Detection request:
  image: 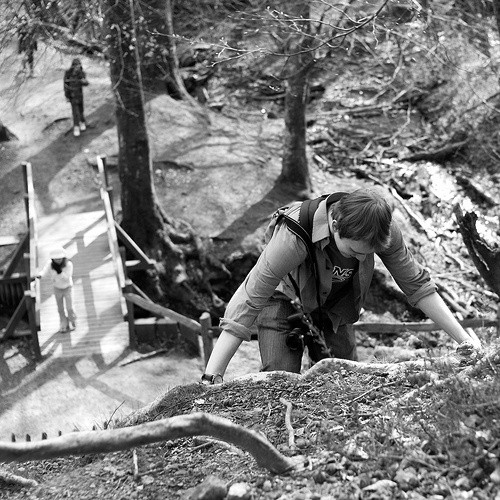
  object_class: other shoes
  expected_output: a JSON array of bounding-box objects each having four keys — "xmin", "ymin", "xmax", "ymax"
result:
[
  {"xmin": 79, "ymin": 122, "xmax": 86, "ymax": 131},
  {"xmin": 60, "ymin": 328, "xmax": 66, "ymax": 332},
  {"xmin": 70, "ymin": 320, "xmax": 75, "ymax": 326},
  {"xmin": 74, "ymin": 126, "xmax": 80, "ymax": 136}
]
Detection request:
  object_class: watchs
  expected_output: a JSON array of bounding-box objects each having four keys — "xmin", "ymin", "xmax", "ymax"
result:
[{"xmin": 202, "ymin": 374, "xmax": 224, "ymax": 384}]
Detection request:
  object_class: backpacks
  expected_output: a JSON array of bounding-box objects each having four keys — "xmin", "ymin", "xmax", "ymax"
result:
[{"xmin": 265, "ymin": 193, "xmax": 329, "ymax": 281}]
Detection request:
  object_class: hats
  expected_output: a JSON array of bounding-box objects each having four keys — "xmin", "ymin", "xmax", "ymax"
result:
[{"xmin": 50, "ymin": 248, "xmax": 66, "ymax": 258}]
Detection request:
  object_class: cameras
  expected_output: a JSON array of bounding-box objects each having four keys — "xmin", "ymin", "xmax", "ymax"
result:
[{"xmin": 286, "ymin": 313, "xmax": 318, "ymax": 352}]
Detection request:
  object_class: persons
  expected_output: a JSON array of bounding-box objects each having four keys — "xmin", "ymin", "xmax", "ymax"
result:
[
  {"xmin": 202, "ymin": 189, "xmax": 481, "ymax": 385},
  {"xmin": 16, "ymin": 29, "xmax": 39, "ymax": 78},
  {"xmin": 33, "ymin": 250, "xmax": 80, "ymax": 332},
  {"xmin": 64, "ymin": 58, "xmax": 90, "ymax": 136}
]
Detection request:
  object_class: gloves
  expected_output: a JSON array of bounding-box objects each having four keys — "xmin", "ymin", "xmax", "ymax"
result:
[{"xmin": 51, "ymin": 260, "xmax": 61, "ymax": 274}]
